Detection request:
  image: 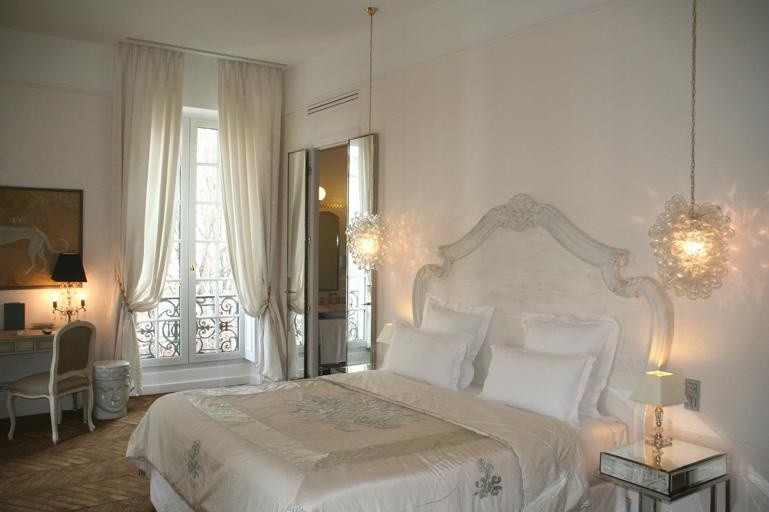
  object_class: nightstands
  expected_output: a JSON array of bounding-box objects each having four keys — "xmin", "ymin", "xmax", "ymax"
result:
[{"xmin": 593, "ymin": 436, "xmax": 732, "ymax": 512}]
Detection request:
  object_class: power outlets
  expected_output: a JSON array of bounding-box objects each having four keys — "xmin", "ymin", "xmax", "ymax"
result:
[{"xmin": 683, "ymin": 378, "xmax": 701, "ymax": 412}]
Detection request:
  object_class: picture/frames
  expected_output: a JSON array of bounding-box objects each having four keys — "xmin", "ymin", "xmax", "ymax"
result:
[{"xmin": 0, "ymin": 186, "xmax": 84, "ymax": 289}]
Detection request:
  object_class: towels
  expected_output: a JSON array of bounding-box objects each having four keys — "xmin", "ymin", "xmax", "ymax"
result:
[{"xmin": 318, "ymin": 318, "xmax": 347, "ymax": 364}]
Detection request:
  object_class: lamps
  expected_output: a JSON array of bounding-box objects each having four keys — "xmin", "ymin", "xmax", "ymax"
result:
[
  {"xmin": 376, "ymin": 324, "xmax": 394, "ymax": 343},
  {"xmin": 345, "ymin": 6, "xmax": 391, "ymax": 273},
  {"xmin": 629, "ymin": 370, "xmax": 688, "ymax": 465},
  {"xmin": 648, "ymin": 1, "xmax": 736, "ymax": 301},
  {"xmin": 50, "ymin": 252, "xmax": 87, "ymax": 410},
  {"xmin": 319, "ymin": 186, "xmax": 326, "ymax": 200}
]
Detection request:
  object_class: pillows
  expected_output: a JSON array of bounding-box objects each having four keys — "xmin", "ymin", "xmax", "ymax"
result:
[
  {"xmin": 474, "ymin": 344, "xmax": 597, "ymax": 429},
  {"xmin": 381, "ymin": 318, "xmax": 478, "ymax": 392},
  {"xmin": 520, "ymin": 311, "xmax": 620, "ymax": 421},
  {"xmin": 420, "ymin": 293, "xmax": 495, "ymax": 390}
]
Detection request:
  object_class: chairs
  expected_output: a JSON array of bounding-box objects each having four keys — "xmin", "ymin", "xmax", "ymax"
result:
[{"xmin": 6, "ymin": 321, "xmax": 96, "ymax": 445}]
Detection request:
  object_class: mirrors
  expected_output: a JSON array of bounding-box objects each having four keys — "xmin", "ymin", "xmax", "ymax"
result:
[
  {"xmin": 287, "ymin": 149, "xmax": 305, "ymax": 380},
  {"xmin": 319, "ymin": 212, "xmax": 339, "ymax": 291},
  {"xmin": 348, "ymin": 133, "xmax": 378, "ymax": 372}
]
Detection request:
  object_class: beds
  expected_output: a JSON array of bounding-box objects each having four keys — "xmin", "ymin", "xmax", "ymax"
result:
[{"xmin": 126, "ymin": 193, "xmax": 674, "ymax": 512}]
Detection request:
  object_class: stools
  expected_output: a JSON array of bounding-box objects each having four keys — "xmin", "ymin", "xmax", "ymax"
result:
[{"xmin": 93, "ymin": 360, "xmax": 132, "ymax": 420}]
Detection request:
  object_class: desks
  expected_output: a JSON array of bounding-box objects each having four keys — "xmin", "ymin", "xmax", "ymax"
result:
[{"xmin": 0, "ymin": 329, "xmax": 88, "ymax": 425}]
